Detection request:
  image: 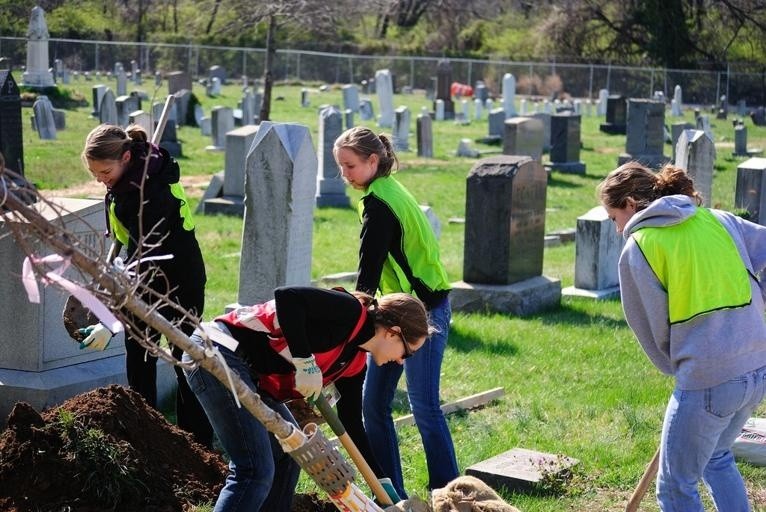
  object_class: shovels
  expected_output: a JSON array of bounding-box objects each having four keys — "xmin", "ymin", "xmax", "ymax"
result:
[{"xmin": 63, "ymin": 94, "xmax": 176, "ymax": 344}]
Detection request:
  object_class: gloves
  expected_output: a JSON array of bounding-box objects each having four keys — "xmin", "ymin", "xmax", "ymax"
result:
[
  {"xmin": 79, "ymin": 322, "xmax": 113, "ymax": 352},
  {"xmin": 292, "ymin": 354, "xmax": 323, "ymax": 401}
]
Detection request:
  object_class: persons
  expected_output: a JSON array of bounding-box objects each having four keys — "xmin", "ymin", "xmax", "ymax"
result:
[
  {"xmin": 331, "ymin": 126, "xmax": 460, "ymax": 499},
  {"xmin": 73, "ymin": 121, "xmax": 208, "ymax": 432},
  {"xmin": 597, "ymin": 159, "xmax": 766, "ymax": 511},
  {"xmin": 181, "ymin": 285, "xmax": 432, "ymax": 511}
]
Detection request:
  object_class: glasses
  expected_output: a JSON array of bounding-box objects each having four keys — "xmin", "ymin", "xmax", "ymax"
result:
[{"xmin": 401, "ymin": 331, "xmax": 414, "ymax": 359}]
